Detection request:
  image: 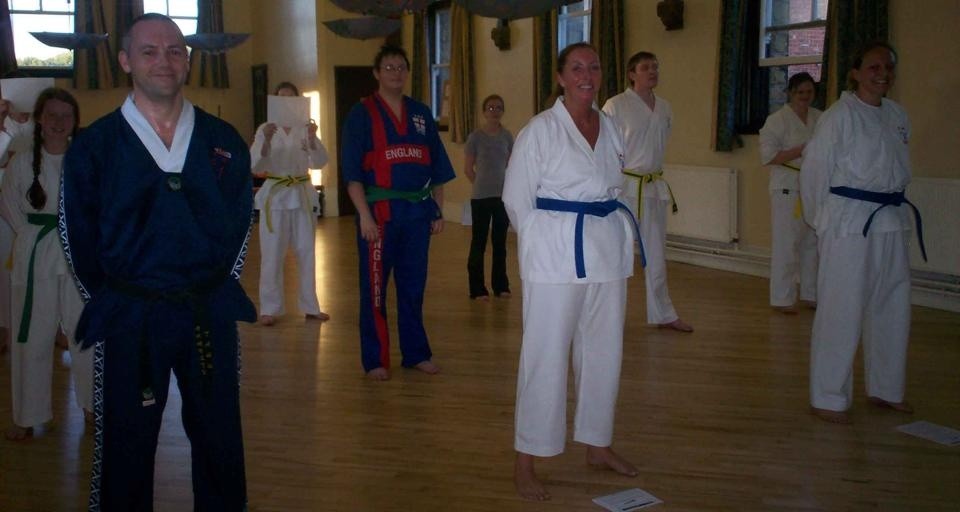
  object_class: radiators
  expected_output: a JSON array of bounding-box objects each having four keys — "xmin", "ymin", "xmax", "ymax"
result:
[
  {"xmin": 663, "ymin": 165, "xmax": 738, "ymax": 250},
  {"xmin": 904, "ymin": 176, "xmax": 960, "ymax": 293}
]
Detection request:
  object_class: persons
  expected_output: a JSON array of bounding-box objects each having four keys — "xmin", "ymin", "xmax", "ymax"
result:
[
  {"xmin": 249, "ymin": 80, "xmax": 329, "ymax": 326},
  {"xmin": 799, "ymin": 39, "xmax": 915, "ymax": 424},
  {"xmin": 603, "ymin": 52, "xmax": 695, "ymax": 334},
  {"xmin": 0, "ymin": 94, "xmax": 30, "ymax": 358},
  {"xmin": 59, "ymin": 14, "xmax": 255, "ymax": 511},
  {"xmin": 463, "ymin": 92, "xmax": 514, "ymax": 302},
  {"xmin": 345, "ymin": 42, "xmax": 457, "ymax": 371},
  {"xmin": 758, "ymin": 71, "xmax": 827, "ymax": 314},
  {"xmin": 501, "ymin": 41, "xmax": 638, "ymax": 501},
  {"xmin": 2, "ymin": 89, "xmax": 111, "ymax": 440}
]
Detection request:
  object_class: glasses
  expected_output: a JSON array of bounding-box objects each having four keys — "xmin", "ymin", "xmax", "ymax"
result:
[
  {"xmin": 379, "ymin": 63, "xmax": 406, "ymax": 73},
  {"xmin": 486, "ymin": 106, "xmax": 503, "ymax": 112}
]
